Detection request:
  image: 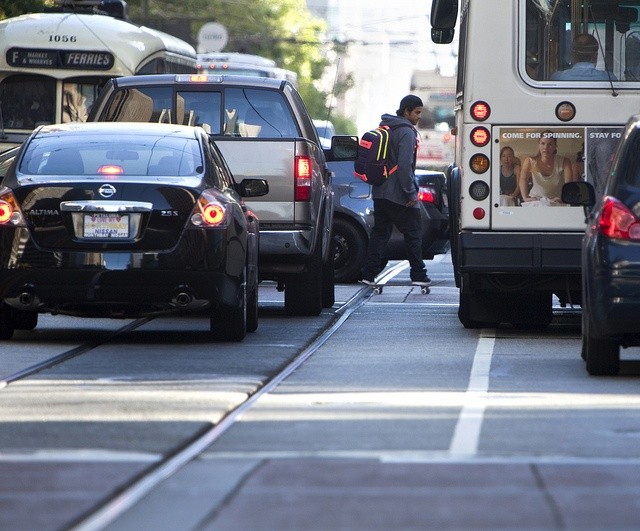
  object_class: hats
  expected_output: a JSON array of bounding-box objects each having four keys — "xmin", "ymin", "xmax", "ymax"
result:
[{"xmin": 400, "ymin": 95, "xmax": 423, "ymax": 111}]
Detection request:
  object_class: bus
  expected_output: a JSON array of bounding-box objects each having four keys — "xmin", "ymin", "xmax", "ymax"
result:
[
  {"xmin": 0, "ymin": 13, "xmax": 197, "ymax": 178},
  {"xmin": 410, "ymin": 70, "xmax": 455, "ymax": 173},
  {"xmin": 431, "ymin": 1, "xmax": 640, "ymax": 331}
]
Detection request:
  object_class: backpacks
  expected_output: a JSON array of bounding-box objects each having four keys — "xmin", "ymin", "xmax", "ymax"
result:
[{"xmin": 354, "ymin": 124, "xmax": 422, "ymax": 187}]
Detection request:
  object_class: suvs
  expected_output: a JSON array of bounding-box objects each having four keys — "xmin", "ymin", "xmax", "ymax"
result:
[{"xmin": 86, "ymin": 75, "xmax": 360, "ymax": 317}]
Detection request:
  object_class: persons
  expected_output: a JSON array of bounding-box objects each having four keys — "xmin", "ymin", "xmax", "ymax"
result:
[
  {"xmin": 517, "ymin": 129, "xmax": 573, "ymax": 207},
  {"xmin": 359, "ymin": 94, "xmax": 431, "ymax": 283},
  {"xmin": 546, "ymin": 33, "xmax": 618, "ymax": 82},
  {"xmin": 500, "ymin": 145, "xmax": 521, "ymax": 201}
]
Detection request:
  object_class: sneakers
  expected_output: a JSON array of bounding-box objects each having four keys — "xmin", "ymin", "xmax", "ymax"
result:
[
  {"xmin": 411, "ymin": 275, "xmax": 431, "ymax": 285},
  {"xmin": 362, "ymin": 274, "xmax": 375, "ymax": 285}
]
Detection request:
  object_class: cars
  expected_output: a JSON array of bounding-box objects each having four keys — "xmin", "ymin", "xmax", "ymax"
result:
[
  {"xmin": 560, "ymin": 115, "xmax": 639, "ymax": 375},
  {"xmin": 0, "ymin": 122, "xmax": 260, "ymax": 343},
  {"xmin": 322, "ymin": 146, "xmax": 449, "ymax": 284}
]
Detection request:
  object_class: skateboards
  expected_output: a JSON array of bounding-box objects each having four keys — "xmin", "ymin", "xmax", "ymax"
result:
[{"xmin": 358, "ymin": 279, "xmax": 432, "ymax": 294}]
